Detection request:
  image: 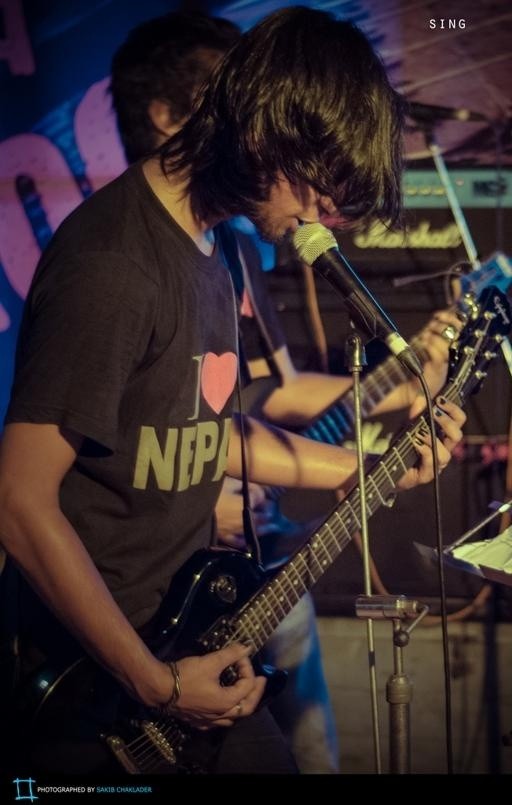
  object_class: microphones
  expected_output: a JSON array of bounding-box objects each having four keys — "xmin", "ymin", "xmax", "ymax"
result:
[
  {"xmin": 292, "ymin": 222, "xmax": 424, "ymax": 377},
  {"xmin": 354, "ymin": 594, "xmax": 428, "ymax": 647},
  {"xmin": 407, "ymin": 98, "xmax": 469, "ymax": 123}
]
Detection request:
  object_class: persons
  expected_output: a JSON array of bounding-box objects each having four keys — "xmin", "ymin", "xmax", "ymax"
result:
[
  {"xmin": 1, "ymin": 7, "xmax": 470, "ymax": 775},
  {"xmin": 102, "ymin": 13, "xmax": 462, "ymax": 775}
]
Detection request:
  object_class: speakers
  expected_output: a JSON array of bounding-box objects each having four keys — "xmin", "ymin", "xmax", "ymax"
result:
[{"xmin": 260, "ymin": 166, "xmax": 512, "ymax": 617}]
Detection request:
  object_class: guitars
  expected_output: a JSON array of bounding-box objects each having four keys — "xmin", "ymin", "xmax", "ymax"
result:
[
  {"xmin": 34, "ymin": 283, "xmax": 510, "ymax": 777},
  {"xmin": 220, "ymin": 290, "xmax": 481, "ymax": 536}
]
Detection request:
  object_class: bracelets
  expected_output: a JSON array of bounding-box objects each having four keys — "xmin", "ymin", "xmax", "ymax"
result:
[{"xmin": 152, "ymin": 660, "xmax": 182, "ymax": 715}]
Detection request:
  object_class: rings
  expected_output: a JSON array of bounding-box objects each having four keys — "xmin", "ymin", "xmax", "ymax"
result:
[
  {"xmin": 440, "ymin": 325, "xmax": 456, "ymax": 340},
  {"xmin": 236, "ymin": 702, "xmax": 243, "ymax": 714}
]
